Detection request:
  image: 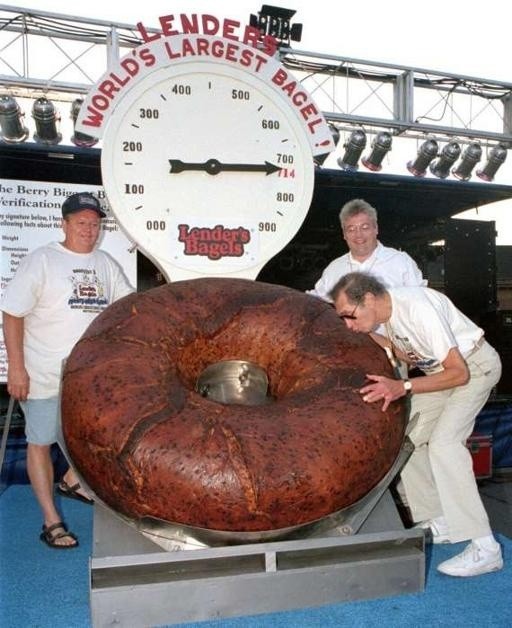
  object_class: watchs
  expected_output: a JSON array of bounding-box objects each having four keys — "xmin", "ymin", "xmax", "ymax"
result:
[{"xmin": 404, "ymin": 378, "xmax": 412, "ymax": 395}]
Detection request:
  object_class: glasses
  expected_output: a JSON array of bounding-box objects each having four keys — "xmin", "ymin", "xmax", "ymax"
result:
[{"xmin": 339, "ymin": 304, "xmax": 358, "ymax": 319}]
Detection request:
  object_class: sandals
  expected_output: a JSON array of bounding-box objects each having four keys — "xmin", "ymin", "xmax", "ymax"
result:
[
  {"xmin": 56, "ymin": 482, "xmax": 94, "ymax": 505},
  {"xmin": 40, "ymin": 522, "xmax": 79, "ymax": 548}
]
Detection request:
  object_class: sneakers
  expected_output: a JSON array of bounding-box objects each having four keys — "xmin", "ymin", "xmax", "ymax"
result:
[
  {"xmin": 437, "ymin": 543, "xmax": 503, "ymax": 577},
  {"xmin": 413, "ymin": 519, "xmax": 450, "ymax": 544}
]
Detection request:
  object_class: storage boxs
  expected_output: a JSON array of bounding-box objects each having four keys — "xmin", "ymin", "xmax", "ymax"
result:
[{"xmin": 461, "ymin": 432, "xmax": 494, "ymax": 486}]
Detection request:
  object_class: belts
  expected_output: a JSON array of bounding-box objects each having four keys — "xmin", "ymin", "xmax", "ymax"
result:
[{"xmin": 473, "ymin": 337, "xmax": 484, "ymax": 352}]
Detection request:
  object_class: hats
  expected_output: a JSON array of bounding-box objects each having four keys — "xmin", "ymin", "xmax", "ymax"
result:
[{"xmin": 62, "ymin": 192, "xmax": 106, "ymax": 217}]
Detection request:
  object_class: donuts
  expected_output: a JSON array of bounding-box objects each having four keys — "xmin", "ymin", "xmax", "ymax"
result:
[{"xmin": 61, "ymin": 277, "xmax": 407, "ymax": 532}]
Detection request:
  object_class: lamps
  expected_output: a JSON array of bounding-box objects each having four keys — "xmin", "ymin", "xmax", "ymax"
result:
[
  {"xmin": 310, "ymin": 117, "xmax": 510, "ymax": 188},
  {"xmin": 0, "ymin": 87, "xmax": 101, "ymax": 152}
]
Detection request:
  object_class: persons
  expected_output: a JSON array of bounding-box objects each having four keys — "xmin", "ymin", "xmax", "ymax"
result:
[
  {"xmin": 1, "ymin": 193, "xmax": 137, "ymax": 549},
  {"xmin": 305, "ymin": 198, "xmax": 429, "ymax": 525},
  {"xmin": 329, "ymin": 273, "xmax": 505, "ymax": 578}
]
